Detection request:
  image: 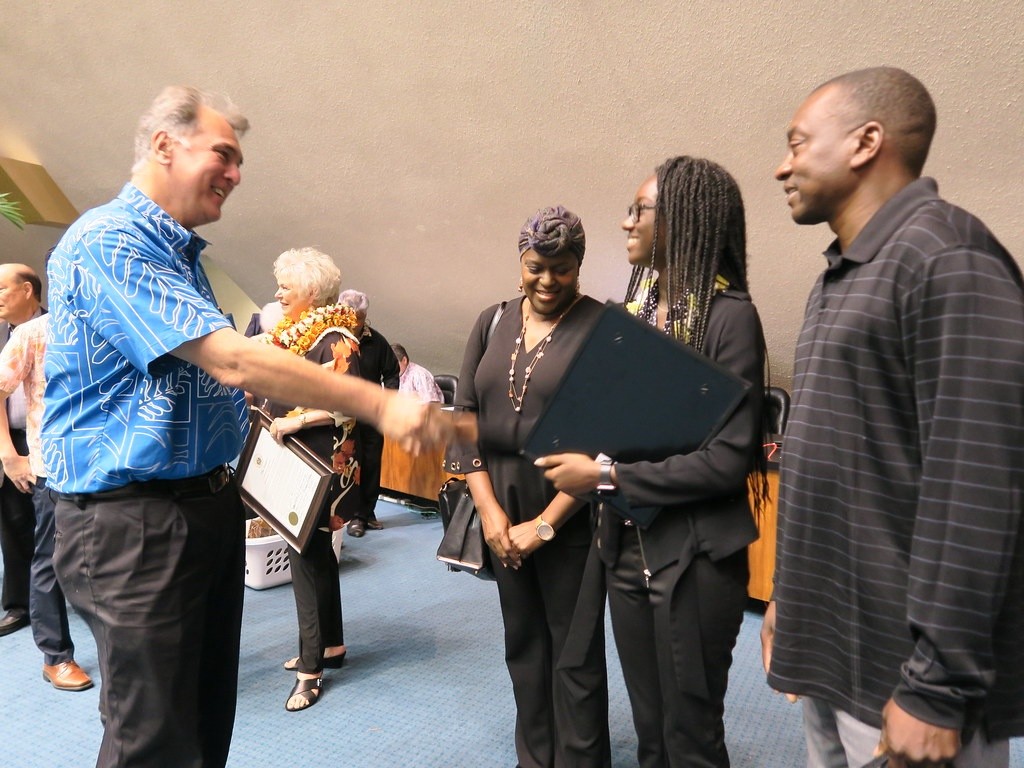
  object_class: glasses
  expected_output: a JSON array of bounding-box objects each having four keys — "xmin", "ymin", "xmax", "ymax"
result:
[{"xmin": 627, "ymin": 202, "xmax": 656, "ymax": 223}]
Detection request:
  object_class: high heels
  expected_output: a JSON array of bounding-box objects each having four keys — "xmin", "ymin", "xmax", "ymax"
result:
[
  {"xmin": 283, "ymin": 650, "xmax": 346, "ymax": 671},
  {"xmin": 285, "ymin": 669, "xmax": 324, "ymax": 712}
]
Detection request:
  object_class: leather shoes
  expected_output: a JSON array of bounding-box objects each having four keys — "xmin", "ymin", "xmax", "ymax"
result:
[
  {"xmin": 367, "ymin": 520, "xmax": 383, "ymax": 529},
  {"xmin": 43, "ymin": 659, "xmax": 93, "ymax": 691},
  {"xmin": 346, "ymin": 518, "xmax": 365, "ymax": 537},
  {"xmin": 0, "ymin": 609, "xmax": 30, "ymax": 635}
]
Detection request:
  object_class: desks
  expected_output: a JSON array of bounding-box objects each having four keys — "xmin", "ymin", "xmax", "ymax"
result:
[{"xmin": 380, "ymin": 421, "xmax": 780, "ymax": 608}]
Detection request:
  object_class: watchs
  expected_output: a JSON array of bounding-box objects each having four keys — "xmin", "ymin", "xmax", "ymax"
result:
[
  {"xmin": 535, "ymin": 515, "xmax": 556, "ymax": 541},
  {"xmin": 596, "ymin": 460, "xmax": 617, "ymax": 494}
]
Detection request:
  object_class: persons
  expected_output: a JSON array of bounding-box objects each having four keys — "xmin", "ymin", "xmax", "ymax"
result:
[
  {"xmin": 42, "ymin": 86, "xmax": 441, "ymax": 768},
  {"xmin": 391, "ymin": 343, "xmax": 444, "ymax": 405},
  {"xmin": 454, "ymin": 205, "xmax": 611, "ymax": 768},
  {"xmin": 760, "ymin": 67, "xmax": 1024, "ymax": 768},
  {"xmin": 0, "ymin": 311, "xmax": 94, "ymax": 691},
  {"xmin": 404, "ymin": 155, "xmax": 766, "ymax": 768},
  {"xmin": 338, "ymin": 290, "xmax": 400, "ymax": 537},
  {"xmin": 250, "ymin": 247, "xmax": 360, "ymax": 713},
  {"xmin": 0, "ymin": 263, "xmax": 48, "ymax": 635}
]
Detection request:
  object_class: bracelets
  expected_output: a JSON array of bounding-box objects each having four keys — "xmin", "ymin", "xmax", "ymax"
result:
[{"xmin": 299, "ymin": 414, "xmax": 308, "ymax": 429}]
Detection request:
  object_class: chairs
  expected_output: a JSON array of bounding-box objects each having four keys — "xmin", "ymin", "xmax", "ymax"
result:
[
  {"xmin": 765, "ymin": 386, "xmax": 789, "ymax": 438},
  {"xmin": 434, "ymin": 373, "xmax": 458, "ymax": 405}
]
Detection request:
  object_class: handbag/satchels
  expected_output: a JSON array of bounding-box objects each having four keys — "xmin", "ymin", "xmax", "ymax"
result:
[
  {"xmin": 436, "ymin": 487, "xmax": 497, "ymax": 581},
  {"xmin": 437, "ymin": 477, "xmax": 467, "ymax": 573}
]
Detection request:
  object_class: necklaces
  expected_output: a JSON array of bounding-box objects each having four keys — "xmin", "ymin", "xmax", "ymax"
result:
[
  {"xmin": 261, "ymin": 304, "xmax": 357, "ymax": 357},
  {"xmin": 509, "ymin": 312, "xmax": 565, "ymax": 412}
]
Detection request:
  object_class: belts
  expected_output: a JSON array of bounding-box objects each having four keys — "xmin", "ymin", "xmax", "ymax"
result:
[{"xmin": 58, "ymin": 464, "xmax": 229, "ymax": 501}]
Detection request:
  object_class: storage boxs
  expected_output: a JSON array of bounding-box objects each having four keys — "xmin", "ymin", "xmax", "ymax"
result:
[{"xmin": 244, "ymin": 515, "xmax": 351, "ymax": 589}]
